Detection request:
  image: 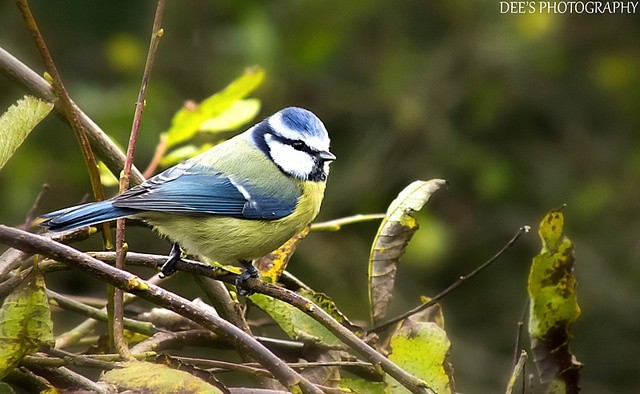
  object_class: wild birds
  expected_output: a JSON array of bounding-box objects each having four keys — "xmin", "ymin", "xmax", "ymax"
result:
[{"xmin": 40, "ymin": 106, "xmax": 339, "ymax": 297}]
